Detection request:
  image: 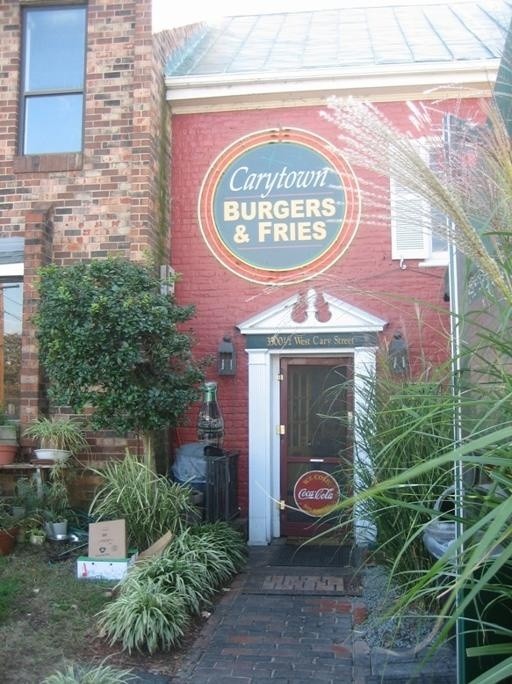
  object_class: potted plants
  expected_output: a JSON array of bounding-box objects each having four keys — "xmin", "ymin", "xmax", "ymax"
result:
[{"xmin": 0, "ymin": 406, "xmax": 96, "ymax": 556}]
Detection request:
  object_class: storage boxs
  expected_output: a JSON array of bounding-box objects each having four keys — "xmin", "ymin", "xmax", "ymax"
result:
[{"xmin": 77, "ymin": 518, "xmax": 139, "ymax": 581}]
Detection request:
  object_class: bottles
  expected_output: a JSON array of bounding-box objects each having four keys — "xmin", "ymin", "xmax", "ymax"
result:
[{"xmin": 198, "ymin": 383, "xmax": 224, "ymax": 447}]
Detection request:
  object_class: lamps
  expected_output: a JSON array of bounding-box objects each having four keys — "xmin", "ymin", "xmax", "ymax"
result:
[
  {"xmin": 388, "ymin": 331, "xmax": 409, "ymax": 373},
  {"xmin": 217, "ymin": 335, "xmax": 237, "ymax": 376}
]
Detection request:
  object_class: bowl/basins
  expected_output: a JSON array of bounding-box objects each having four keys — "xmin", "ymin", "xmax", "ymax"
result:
[{"xmin": 47, "ymin": 534, "xmax": 89, "ymax": 546}]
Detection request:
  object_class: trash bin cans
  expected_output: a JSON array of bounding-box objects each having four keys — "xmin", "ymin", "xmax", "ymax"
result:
[{"xmin": 175, "ymin": 442, "xmax": 227, "ymax": 526}]
